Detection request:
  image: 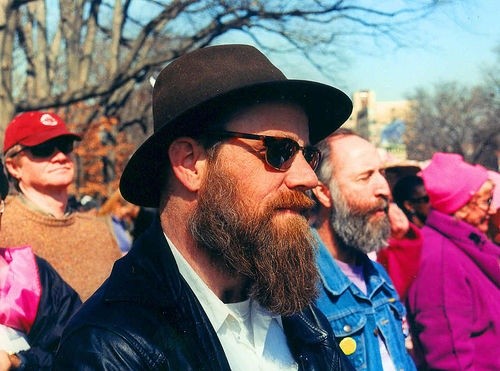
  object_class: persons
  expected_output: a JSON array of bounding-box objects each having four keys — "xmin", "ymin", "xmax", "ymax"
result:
[
  {"xmin": 306, "ymin": 127, "xmax": 417, "ymax": 371},
  {"xmin": 90, "ymin": 155, "xmax": 431, "ymax": 299},
  {"xmin": 0, "ymin": 112, "xmax": 122, "ymax": 304},
  {"xmin": 54, "ymin": 44, "xmax": 358, "ymax": 371},
  {"xmin": 0, "ymin": 158, "xmax": 84, "ymax": 371},
  {"xmin": 408, "ymin": 152, "xmax": 500, "ymax": 371}
]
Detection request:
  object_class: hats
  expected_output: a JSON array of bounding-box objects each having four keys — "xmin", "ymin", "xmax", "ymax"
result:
[
  {"xmin": 416, "ymin": 152, "xmax": 488, "ymax": 215},
  {"xmin": 2, "ymin": 111, "xmax": 82, "ymax": 153},
  {"xmin": 119, "ymin": 44, "xmax": 353, "ymax": 208}
]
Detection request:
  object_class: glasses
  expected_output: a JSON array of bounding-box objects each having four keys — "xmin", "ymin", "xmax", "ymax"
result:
[
  {"xmin": 9, "ymin": 136, "xmax": 73, "ymax": 158},
  {"xmin": 202, "ymin": 130, "xmax": 321, "ymax": 172},
  {"xmin": 409, "ymin": 194, "xmax": 430, "ymax": 204}
]
